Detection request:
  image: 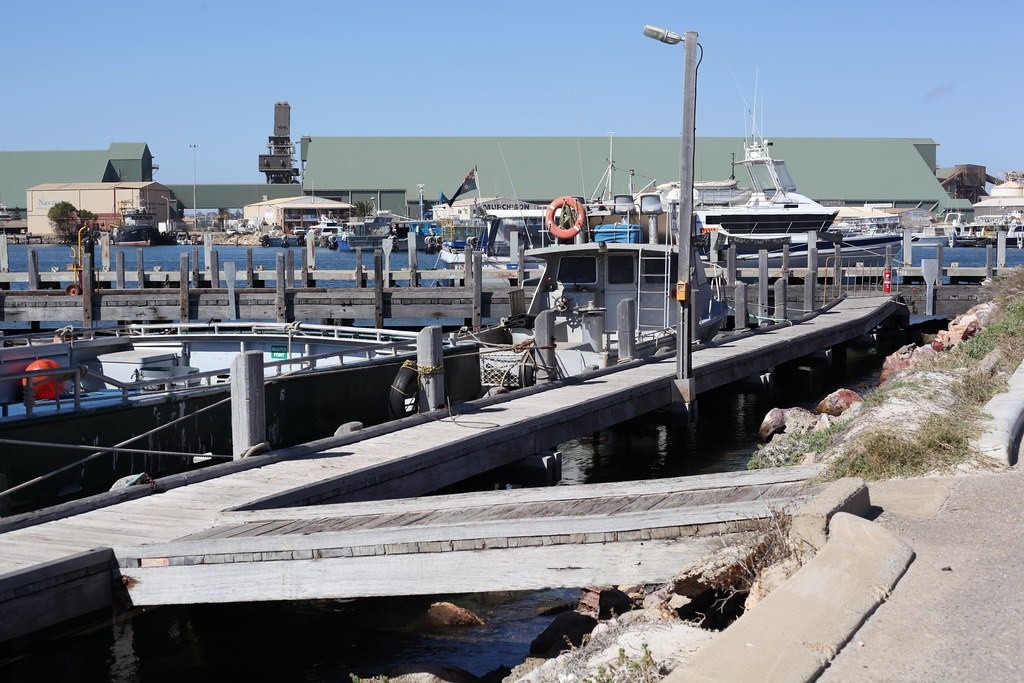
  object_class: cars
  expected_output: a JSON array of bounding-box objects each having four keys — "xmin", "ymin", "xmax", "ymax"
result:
[{"xmin": 226, "ymin": 229, "xmax": 236, "ymax": 235}]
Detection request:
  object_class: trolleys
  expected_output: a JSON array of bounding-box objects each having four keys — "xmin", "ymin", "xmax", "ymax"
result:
[{"xmin": 66, "ymin": 221, "xmax": 87, "ymax": 297}]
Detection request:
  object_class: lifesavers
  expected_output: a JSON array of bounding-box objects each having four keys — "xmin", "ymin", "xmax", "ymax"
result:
[
  {"xmin": 546, "ymin": 195, "xmax": 586, "ymax": 237},
  {"xmin": 388, "ymin": 362, "xmax": 421, "ymax": 417}
]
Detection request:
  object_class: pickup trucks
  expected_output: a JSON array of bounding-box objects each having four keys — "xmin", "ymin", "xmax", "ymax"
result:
[
  {"xmin": 272, "ymin": 225, "xmax": 283, "ymax": 233},
  {"xmin": 291, "ymin": 227, "xmax": 305, "ymax": 235}
]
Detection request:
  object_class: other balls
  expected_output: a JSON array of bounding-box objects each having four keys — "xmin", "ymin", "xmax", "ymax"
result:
[{"xmin": 24, "ymin": 359, "xmax": 64, "ymax": 401}]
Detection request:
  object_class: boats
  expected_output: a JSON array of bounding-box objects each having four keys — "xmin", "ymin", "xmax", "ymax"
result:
[
  {"xmin": 900, "ymin": 212, "xmax": 970, "ymax": 247},
  {"xmin": 961, "ymin": 210, "xmax": 1024, "ymax": 249},
  {"xmin": 0, "ymin": 322, "xmax": 482, "ymax": 517},
  {"xmin": 109, "ymin": 200, "xmax": 160, "ymax": 246},
  {"xmin": 446, "ymin": 136, "xmax": 731, "ymax": 398},
  {"xmin": 439, "ymin": 140, "xmax": 920, "ymax": 286},
  {"xmin": 260, "ymin": 215, "xmax": 439, "ymax": 253},
  {"xmin": 176, "ymin": 232, "xmax": 188, "ymax": 246}
]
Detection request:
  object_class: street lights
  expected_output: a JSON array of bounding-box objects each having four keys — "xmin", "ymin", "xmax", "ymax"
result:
[
  {"xmin": 417, "ymin": 184, "xmax": 425, "ymax": 220},
  {"xmin": 643, "ymin": 26, "xmax": 697, "ymax": 381},
  {"xmin": 161, "ymin": 196, "xmax": 170, "ymax": 231},
  {"xmin": 190, "ymin": 144, "xmax": 200, "ymax": 229}
]
